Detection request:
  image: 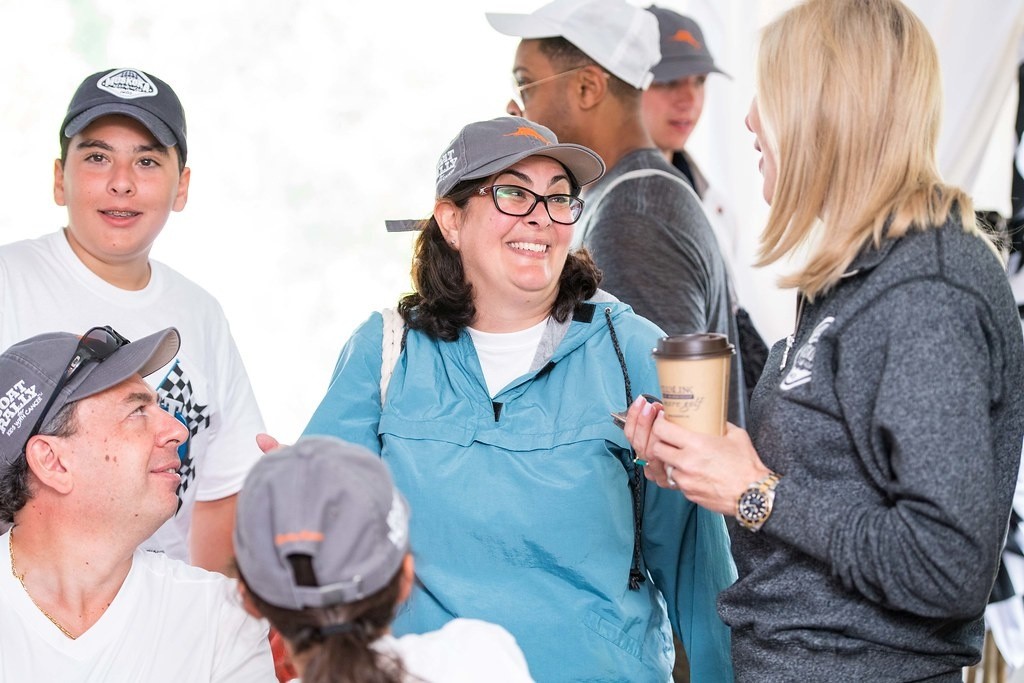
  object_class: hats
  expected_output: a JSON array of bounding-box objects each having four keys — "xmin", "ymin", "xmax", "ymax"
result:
[
  {"xmin": 643, "ymin": 4, "xmax": 730, "ymax": 84},
  {"xmin": 0, "ymin": 328, "xmax": 184, "ymax": 479},
  {"xmin": 436, "ymin": 117, "xmax": 606, "ymax": 199},
  {"xmin": 486, "ymin": 0, "xmax": 663, "ymax": 91},
  {"xmin": 232, "ymin": 435, "xmax": 409, "ymax": 610},
  {"xmin": 60, "ymin": 69, "xmax": 188, "ymax": 168}
]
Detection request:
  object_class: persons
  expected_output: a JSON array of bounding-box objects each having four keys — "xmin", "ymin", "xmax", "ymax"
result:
[
  {"xmin": 0, "ymin": 326, "xmax": 278, "ymax": 682},
  {"xmin": 486, "ymin": 0, "xmax": 747, "ymax": 429},
  {"xmin": 640, "ymin": 6, "xmax": 768, "ymax": 386},
  {"xmin": 232, "ymin": 436, "xmax": 532, "ymax": 682},
  {"xmin": 256, "ymin": 117, "xmax": 741, "ymax": 683},
  {"xmin": 624, "ymin": 0, "xmax": 1024, "ymax": 683},
  {"xmin": 1, "ymin": 66, "xmax": 267, "ymax": 576}
]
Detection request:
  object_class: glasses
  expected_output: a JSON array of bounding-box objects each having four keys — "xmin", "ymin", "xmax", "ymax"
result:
[
  {"xmin": 511, "ymin": 65, "xmax": 610, "ymax": 112},
  {"xmin": 454, "ymin": 186, "xmax": 584, "ymax": 225},
  {"xmin": 21, "ymin": 325, "xmax": 131, "ymax": 455}
]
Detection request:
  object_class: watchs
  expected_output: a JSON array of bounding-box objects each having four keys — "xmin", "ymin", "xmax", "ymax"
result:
[{"xmin": 736, "ymin": 472, "xmax": 783, "ymax": 532}]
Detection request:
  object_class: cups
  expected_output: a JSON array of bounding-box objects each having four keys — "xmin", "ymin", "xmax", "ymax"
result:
[{"xmin": 651, "ymin": 332, "xmax": 737, "ymax": 444}]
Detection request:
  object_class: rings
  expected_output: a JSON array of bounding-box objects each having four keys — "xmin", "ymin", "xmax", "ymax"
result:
[{"xmin": 667, "ymin": 466, "xmax": 677, "ymax": 488}]
